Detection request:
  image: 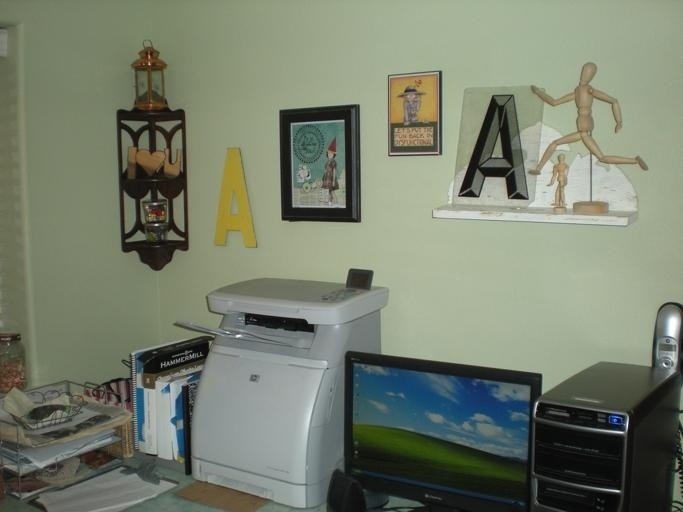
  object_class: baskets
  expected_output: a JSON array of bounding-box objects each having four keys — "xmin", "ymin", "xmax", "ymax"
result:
[{"xmin": 84, "ymin": 361, "xmax": 133, "ymax": 458}]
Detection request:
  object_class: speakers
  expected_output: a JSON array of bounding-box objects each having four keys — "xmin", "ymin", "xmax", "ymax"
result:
[{"xmin": 325, "ymin": 468, "xmax": 387, "ymax": 512}]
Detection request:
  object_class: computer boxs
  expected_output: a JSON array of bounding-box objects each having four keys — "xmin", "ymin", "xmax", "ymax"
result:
[{"xmin": 530, "ymin": 361, "xmax": 678, "ymax": 511}]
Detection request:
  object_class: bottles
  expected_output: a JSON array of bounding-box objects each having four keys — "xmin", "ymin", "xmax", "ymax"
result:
[
  {"xmin": 142, "ymin": 199, "xmax": 169, "ymax": 222},
  {"xmin": 143, "ymin": 223, "xmax": 168, "ymax": 243},
  {"xmin": 0, "ymin": 333, "xmax": 28, "ymax": 392}
]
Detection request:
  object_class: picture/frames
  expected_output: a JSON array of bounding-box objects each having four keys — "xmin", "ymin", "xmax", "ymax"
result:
[
  {"xmin": 277, "ymin": 101, "xmax": 360, "ymax": 225},
  {"xmin": 385, "ymin": 68, "xmax": 445, "ymax": 158}
]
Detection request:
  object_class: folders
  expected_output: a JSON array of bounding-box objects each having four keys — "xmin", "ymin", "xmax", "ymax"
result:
[{"xmin": 28, "ymin": 457, "xmax": 180, "ymax": 512}]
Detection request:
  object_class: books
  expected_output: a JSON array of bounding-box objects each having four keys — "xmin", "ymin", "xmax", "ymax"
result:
[
  {"xmin": 131, "ymin": 335, "xmax": 212, "ymax": 473},
  {"xmin": 34, "ymin": 465, "xmax": 180, "ymax": 512},
  {"xmin": 0, "ymin": 386, "xmax": 132, "ymax": 501}
]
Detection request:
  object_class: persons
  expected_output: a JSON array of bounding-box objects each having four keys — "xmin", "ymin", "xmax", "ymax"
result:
[
  {"xmin": 397, "ymin": 84, "xmax": 426, "ymax": 126},
  {"xmin": 531, "ymin": 62, "xmax": 648, "ymax": 176},
  {"xmin": 321, "ymin": 136, "xmax": 339, "ymax": 205},
  {"xmin": 545, "ymin": 155, "xmax": 570, "ymax": 211}
]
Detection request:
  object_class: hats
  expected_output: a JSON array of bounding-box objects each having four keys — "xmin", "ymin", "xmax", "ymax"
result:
[
  {"xmin": 328, "ymin": 137, "xmax": 336, "ymax": 153},
  {"xmin": 396, "ymin": 87, "xmax": 425, "ymax": 96}
]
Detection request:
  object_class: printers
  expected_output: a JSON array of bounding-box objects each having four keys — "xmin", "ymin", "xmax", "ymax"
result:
[{"xmin": 174, "ymin": 269, "xmax": 388, "ymax": 508}]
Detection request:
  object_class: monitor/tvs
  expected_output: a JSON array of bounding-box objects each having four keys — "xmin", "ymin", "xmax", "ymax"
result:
[{"xmin": 345, "ymin": 350, "xmax": 543, "ymax": 512}]
